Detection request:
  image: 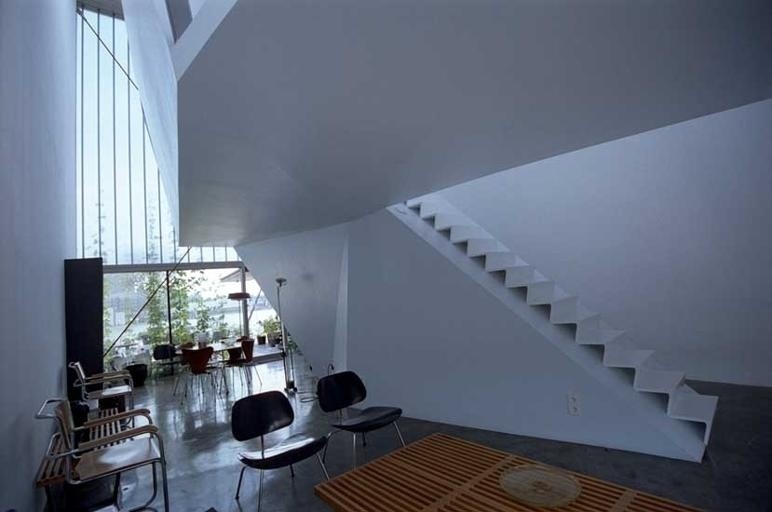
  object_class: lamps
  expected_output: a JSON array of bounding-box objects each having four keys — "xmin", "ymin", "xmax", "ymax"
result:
[{"xmin": 228, "ymin": 293, "xmax": 251, "ymax": 337}]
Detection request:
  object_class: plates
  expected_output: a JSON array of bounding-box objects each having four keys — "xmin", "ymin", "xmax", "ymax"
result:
[{"xmin": 500, "ymin": 463, "xmax": 582, "ymax": 508}]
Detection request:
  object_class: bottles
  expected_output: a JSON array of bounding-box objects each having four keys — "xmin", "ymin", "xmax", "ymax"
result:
[{"xmin": 229, "ymin": 328, "xmax": 235, "ymax": 346}]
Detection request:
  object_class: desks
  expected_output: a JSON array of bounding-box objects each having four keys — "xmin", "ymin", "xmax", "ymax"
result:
[{"xmin": 313, "ymin": 430, "xmax": 697, "ymax": 512}]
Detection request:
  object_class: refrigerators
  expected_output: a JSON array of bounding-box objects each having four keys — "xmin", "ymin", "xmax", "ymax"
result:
[{"xmin": 64, "ymin": 257, "xmax": 104, "ymax": 400}]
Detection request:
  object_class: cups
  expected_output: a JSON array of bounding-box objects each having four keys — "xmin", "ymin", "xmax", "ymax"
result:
[{"xmin": 198, "ymin": 333, "xmax": 208, "ymax": 348}]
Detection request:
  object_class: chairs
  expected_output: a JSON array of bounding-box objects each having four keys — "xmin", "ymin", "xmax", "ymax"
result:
[
  {"xmin": 231, "ymin": 390, "xmax": 330, "ymax": 512},
  {"xmin": 152, "ymin": 336, "xmax": 262, "ymax": 405},
  {"xmin": 36, "ymin": 398, "xmax": 170, "ymax": 512},
  {"xmin": 317, "ymin": 370, "xmax": 406, "ymax": 472},
  {"xmin": 69, "ymin": 361, "xmax": 135, "ymax": 428}
]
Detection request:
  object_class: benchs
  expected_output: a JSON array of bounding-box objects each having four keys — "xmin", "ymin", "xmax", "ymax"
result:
[{"xmin": 36, "ymin": 408, "xmax": 124, "ymax": 512}]
